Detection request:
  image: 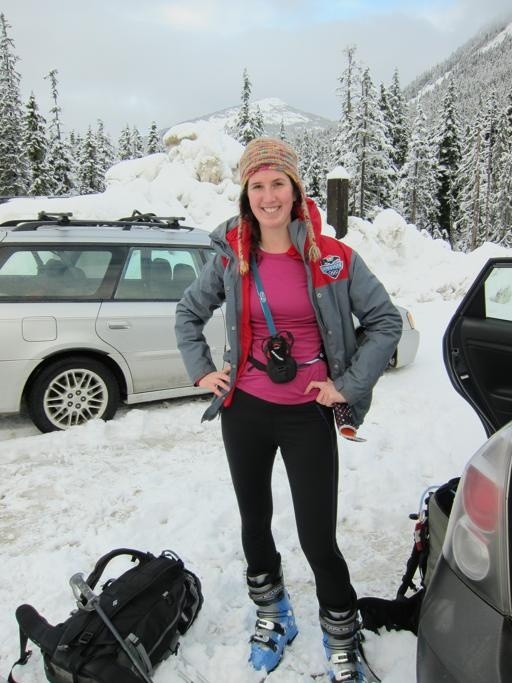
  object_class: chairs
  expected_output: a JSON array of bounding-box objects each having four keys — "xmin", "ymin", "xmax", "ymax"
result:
[
  {"xmin": 172, "ymin": 260, "xmax": 198, "ymax": 299},
  {"xmin": 145, "ymin": 257, "xmax": 174, "ymax": 301}
]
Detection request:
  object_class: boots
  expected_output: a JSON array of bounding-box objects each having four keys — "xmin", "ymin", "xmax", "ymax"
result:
[
  {"xmin": 314, "ymin": 582, "xmax": 371, "ymax": 683},
  {"xmin": 243, "ymin": 551, "xmax": 301, "ymax": 676}
]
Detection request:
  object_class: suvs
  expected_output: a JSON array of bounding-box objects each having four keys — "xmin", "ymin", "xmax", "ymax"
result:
[{"xmin": 0, "ymin": 209, "xmax": 418, "ymax": 433}]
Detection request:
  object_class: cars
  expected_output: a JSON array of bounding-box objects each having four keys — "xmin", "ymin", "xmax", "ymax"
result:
[{"xmin": 413, "ymin": 254, "xmax": 512, "ymax": 682}]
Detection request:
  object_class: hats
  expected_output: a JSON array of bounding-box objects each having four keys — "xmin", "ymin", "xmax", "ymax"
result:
[{"xmin": 234, "ymin": 135, "xmax": 324, "ymax": 277}]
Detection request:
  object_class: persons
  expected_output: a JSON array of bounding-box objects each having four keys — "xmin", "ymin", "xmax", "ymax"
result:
[{"xmin": 173, "ymin": 135, "xmax": 404, "ymax": 683}]
